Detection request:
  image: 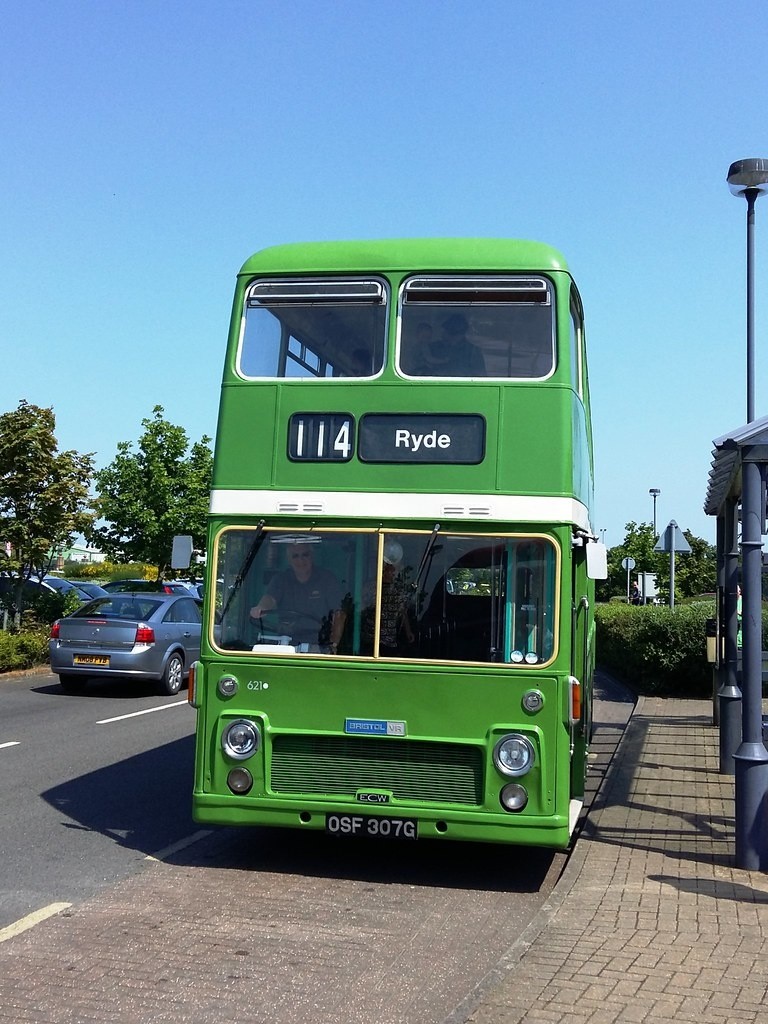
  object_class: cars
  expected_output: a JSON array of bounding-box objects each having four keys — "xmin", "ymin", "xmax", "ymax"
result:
[
  {"xmin": 0, "ymin": 574, "xmax": 110, "ymax": 615},
  {"xmin": 70, "ymin": 580, "xmax": 127, "ymax": 614},
  {"xmin": 48, "ymin": 592, "xmax": 221, "ymax": 695},
  {"xmin": 103, "ymin": 579, "xmax": 193, "ymax": 595}
]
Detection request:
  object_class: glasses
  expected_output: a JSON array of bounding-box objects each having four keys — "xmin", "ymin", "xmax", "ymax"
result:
[{"xmin": 291, "ymin": 550, "xmax": 312, "ymax": 559}]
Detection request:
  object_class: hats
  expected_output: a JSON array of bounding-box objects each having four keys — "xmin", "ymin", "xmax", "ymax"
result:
[
  {"xmin": 441, "ymin": 313, "xmax": 469, "ymax": 331},
  {"xmin": 384, "ymin": 541, "xmax": 404, "ymax": 564}
]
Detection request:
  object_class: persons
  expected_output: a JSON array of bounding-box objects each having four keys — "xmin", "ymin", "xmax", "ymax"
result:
[
  {"xmin": 363, "ymin": 565, "xmax": 414, "ymax": 655},
  {"xmin": 630, "ymin": 581, "xmax": 640, "ymax": 605},
  {"xmin": 249, "ymin": 542, "xmax": 349, "ymax": 652},
  {"xmin": 407, "ymin": 314, "xmax": 487, "ymax": 376}
]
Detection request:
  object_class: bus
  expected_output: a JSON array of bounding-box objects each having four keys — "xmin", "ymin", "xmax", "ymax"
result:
[{"xmin": 170, "ymin": 235, "xmax": 608, "ymax": 852}]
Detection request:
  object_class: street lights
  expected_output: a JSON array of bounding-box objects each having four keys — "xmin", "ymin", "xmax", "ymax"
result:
[
  {"xmin": 727, "ymin": 157, "xmax": 768, "ymax": 423},
  {"xmin": 649, "ymin": 488, "xmax": 661, "ymax": 537}
]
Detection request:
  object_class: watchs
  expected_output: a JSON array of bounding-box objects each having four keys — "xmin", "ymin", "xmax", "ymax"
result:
[{"xmin": 330, "ymin": 642, "xmax": 339, "ymax": 648}]
